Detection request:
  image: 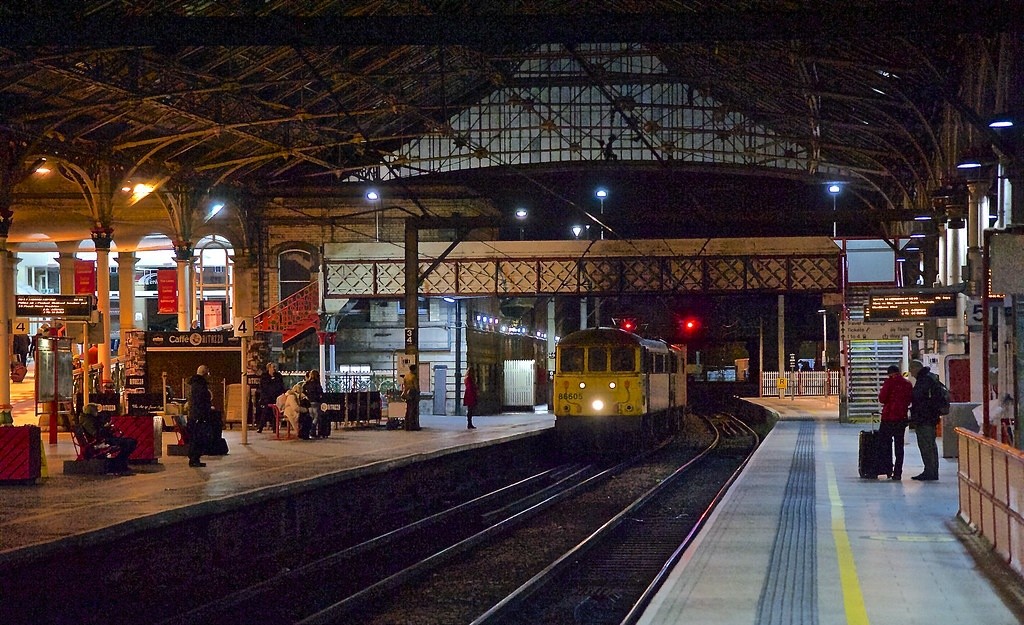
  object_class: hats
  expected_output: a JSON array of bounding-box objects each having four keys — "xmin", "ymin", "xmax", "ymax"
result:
[
  {"xmin": 82, "ymin": 403, "xmax": 98, "ymax": 415},
  {"xmin": 73, "ymin": 355, "xmax": 81, "ymax": 361},
  {"xmin": 409, "ymin": 364, "xmax": 417, "ymax": 372},
  {"xmin": 887, "ymin": 366, "xmax": 900, "ymax": 374},
  {"xmin": 196, "ymin": 365, "xmax": 209, "ymax": 376}
]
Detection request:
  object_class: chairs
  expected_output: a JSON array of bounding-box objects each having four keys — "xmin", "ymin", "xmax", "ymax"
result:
[
  {"xmin": 172, "ymin": 415, "xmax": 189, "ymax": 445},
  {"xmin": 268, "ymin": 403, "xmax": 292, "ymax": 439},
  {"xmin": 69, "ymin": 426, "xmax": 124, "ymax": 477}
]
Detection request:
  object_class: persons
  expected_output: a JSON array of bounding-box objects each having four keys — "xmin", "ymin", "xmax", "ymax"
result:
[
  {"xmin": 878, "ymin": 365, "xmax": 913, "ymax": 481},
  {"xmin": 88, "ymin": 344, "xmax": 98, "ymax": 394},
  {"xmin": 79, "ymin": 403, "xmax": 137, "ymax": 470},
  {"xmin": 29, "ymin": 328, "xmax": 44, "ymax": 358},
  {"xmin": 254, "ymin": 362, "xmax": 284, "ymax": 433},
  {"xmin": 463, "ymin": 366, "xmax": 479, "ymax": 429},
  {"xmin": 404, "ymin": 364, "xmax": 422, "ymax": 431},
  {"xmin": 908, "ymin": 359, "xmax": 939, "ymax": 482},
  {"xmin": 284, "ymin": 369, "xmax": 323, "ymax": 440},
  {"xmin": 190, "ymin": 319, "xmax": 200, "ymax": 332},
  {"xmin": 13, "ymin": 334, "xmax": 30, "ymax": 366},
  {"xmin": 188, "ymin": 365, "xmax": 211, "ymax": 467}
]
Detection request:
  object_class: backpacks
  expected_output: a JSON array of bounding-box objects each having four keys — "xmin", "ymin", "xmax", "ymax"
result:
[{"xmin": 924, "ymin": 374, "xmax": 950, "ymax": 416}]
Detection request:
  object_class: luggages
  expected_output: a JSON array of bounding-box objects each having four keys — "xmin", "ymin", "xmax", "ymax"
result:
[
  {"xmin": 10, "ymin": 357, "xmax": 31, "ymax": 384},
  {"xmin": 858, "ymin": 411, "xmax": 891, "ymax": 478}
]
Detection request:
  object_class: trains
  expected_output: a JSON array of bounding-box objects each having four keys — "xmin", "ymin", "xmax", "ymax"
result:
[{"xmin": 550, "ymin": 326, "xmax": 689, "ymax": 454}]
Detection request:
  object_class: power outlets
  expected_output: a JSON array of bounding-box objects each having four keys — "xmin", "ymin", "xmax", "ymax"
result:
[{"xmin": 956, "ymin": 156, "xmax": 1008, "ymax": 169}]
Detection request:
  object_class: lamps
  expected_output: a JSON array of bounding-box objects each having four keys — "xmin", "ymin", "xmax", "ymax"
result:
[{"xmin": 989, "ymin": 118, "xmax": 1024, "ymax": 127}]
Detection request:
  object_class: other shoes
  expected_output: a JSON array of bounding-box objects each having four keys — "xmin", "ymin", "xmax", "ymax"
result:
[
  {"xmin": 911, "ymin": 473, "xmax": 939, "ymax": 480},
  {"xmin": 273, "ymin": 430, "xmax": 276, "ymax": 433},
  {"xmin": 404, "ymin": 428, "xmax": 410, "ymax": 431},
  {"xmin": 409, "ymin": 427, "xmax": 422, "ymax": 431},
  {"xmin": 189, "ymin": 461, "xmax": 207, "ymax": 467},
  {"xmin": 256, "ymin": 428, "xmax": 262, "ymax": 433},
  {"xmin": 892, "ymin": 475, "xmax": 902, "ymax": 480}
]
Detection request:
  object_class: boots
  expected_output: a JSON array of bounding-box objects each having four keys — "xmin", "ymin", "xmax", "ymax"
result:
[{"xmin": 467, "ymin": 419, "xmax": 477, "ymax": 429}]
